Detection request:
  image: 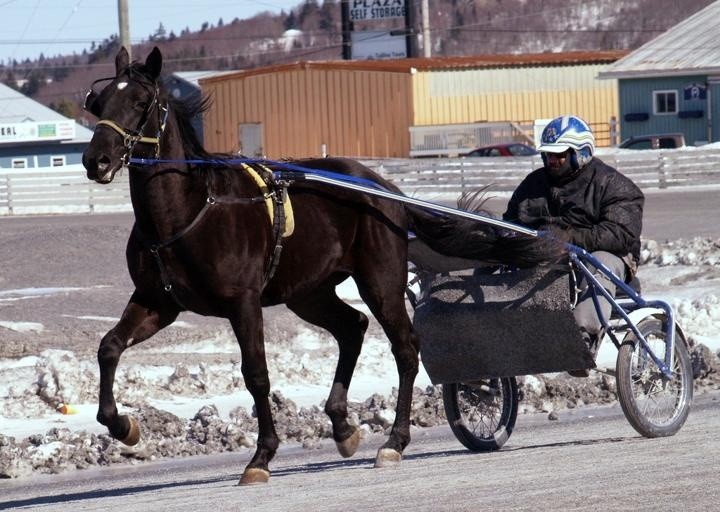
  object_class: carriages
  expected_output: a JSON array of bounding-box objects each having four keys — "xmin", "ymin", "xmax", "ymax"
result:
[{"xmin": 80, "ymin": 45, "xmax": 695, "ymax": 488}]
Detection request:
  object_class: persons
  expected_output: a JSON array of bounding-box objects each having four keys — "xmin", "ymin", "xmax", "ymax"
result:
[{"xmin": 501, "ymin": 113, "xmax": 645, "ymax": 378}]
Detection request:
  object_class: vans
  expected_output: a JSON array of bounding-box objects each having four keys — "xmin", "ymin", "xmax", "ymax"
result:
[{"xmin": 616, "ymin": 134, "xmax": 687, "ymax": 148}]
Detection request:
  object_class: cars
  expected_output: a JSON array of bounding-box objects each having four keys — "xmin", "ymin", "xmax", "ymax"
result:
[{"xmin": 461, "ymin": 142, "xmax": 544, "ymax": 158}]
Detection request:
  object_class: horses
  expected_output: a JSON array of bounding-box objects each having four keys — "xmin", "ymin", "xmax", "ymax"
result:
[{"xmin": 81, "ymin": 46, "xmax": 574, "ymax": 487}]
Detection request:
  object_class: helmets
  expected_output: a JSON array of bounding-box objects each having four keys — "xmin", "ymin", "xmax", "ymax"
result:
[{"xmin": 535, "ymin": 115, "xmax": 595, "ymax": 181}]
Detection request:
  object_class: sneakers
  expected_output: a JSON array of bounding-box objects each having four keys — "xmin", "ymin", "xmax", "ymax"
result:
[{"xmin": 569, "ymin": 368, "xmax": 590, "ymax": 377}]
